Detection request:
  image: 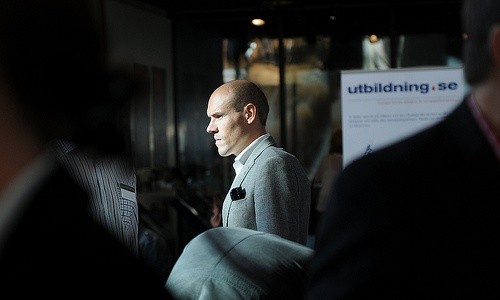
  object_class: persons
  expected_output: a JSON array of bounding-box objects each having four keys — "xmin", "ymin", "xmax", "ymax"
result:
[
  {"xmin": 0, "ymin": 82, "xmax": 149, "ymax": 300},
  {"xmin": 307, "ymin": 0, "xmax": 500, "ymax": 300},
  {"xmin": 164, "ymin": 80, "xmax": 311, "ymax": 243}
]
